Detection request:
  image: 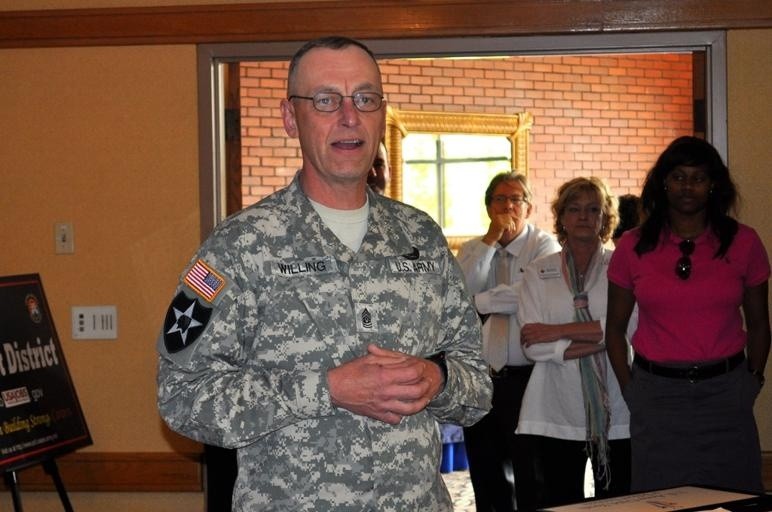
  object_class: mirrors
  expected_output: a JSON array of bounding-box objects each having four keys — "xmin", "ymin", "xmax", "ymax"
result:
[{"xmin": 388, "ymin": 105, "xmax": 537, "ymax": 256}]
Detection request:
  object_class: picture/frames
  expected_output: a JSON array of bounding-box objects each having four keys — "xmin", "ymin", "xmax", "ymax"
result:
[{"xmin": 533, "ymin": 478, "xmax": 765, "ymax": 511}]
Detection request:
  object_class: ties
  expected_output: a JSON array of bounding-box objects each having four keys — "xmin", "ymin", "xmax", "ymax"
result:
[{"xmin": 487, "ymin": 249, "xmax": 511, "ymax": 373}]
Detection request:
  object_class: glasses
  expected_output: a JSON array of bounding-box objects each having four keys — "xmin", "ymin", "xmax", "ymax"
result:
[
  {"xmin": 490, "ymin": 196, "xmax": 526, "ymax": 204},
  {"xmin": 287, "ymin": 90, "xmax": 382, "ymax": 113},
  {"xmin": 675, "ymin": 239, "xmax": 694, "ymax": 281}
]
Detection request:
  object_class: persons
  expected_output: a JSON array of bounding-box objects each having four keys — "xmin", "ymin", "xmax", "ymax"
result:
[
  {"xmin": 455, "ymin": 171, "xmax": 560, "ymax": 512},
  {"xmin": 511, "ymin": 174, "xmax": 639, "ymax": 512},
  {"xmin": 155, "ymin": 35, "xmax": 495, "ymax": 512},
  {"xmin": 604, "ymin": 134, "xmax": 772, "ymax": 497},
  {"xmin": 365, "ymin": 141, "xmax": 391, "ymax": 197}
]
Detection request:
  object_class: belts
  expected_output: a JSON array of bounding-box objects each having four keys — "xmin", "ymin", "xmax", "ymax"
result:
[
  {"xmin": 489, "ymin": 366, "xmax": 534, "ymax": 380},
  {"xmin": 634, "ymin": 352, "xmax": 746, "ymax": 381}
]
